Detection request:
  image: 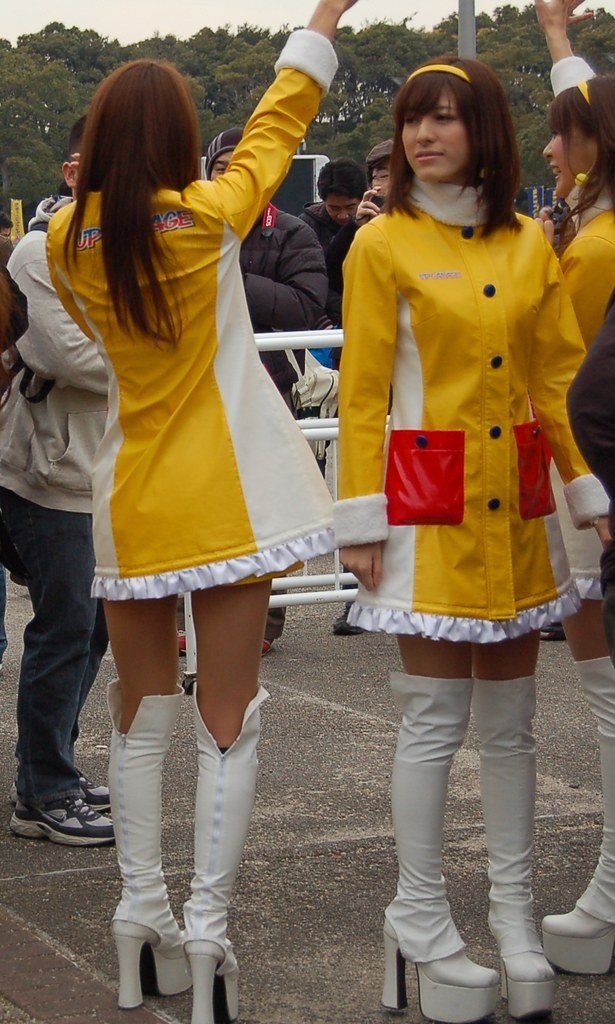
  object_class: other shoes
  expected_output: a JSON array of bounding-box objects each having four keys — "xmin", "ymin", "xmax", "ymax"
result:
[
  {"xmin": 176, "ymin": 629, "xmax": 186, "ymax": 656},
  {"xmin": 333, "ymin": 606, "xmax": 363, "ymax": 634},
  {"xmin": 262, "ymin": 638, "xmax": 275, "ymax": 656},
  {"xmin": 540, "ymin": 622, "xmax": 566, "ymax": 640}
]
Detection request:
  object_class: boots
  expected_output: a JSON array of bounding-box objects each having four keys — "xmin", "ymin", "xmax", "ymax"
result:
[
  {"xmin": 542, "ymin": 657, "xmax": 615, "ymax": 974},
  {"xmin": 107, "ymin": 677, "xmax": 192, "ymax": 1008},
  {"xmin": 474, "ymin": 675, "xmax": 553, "ymax": 1018},
  {"xmin": 182, "ymin": 681, "xmax": 270, "ymax": 1024},
  {"xmin": 383, "ymin": 670, "xmax": 499, "ymax": 1024}
]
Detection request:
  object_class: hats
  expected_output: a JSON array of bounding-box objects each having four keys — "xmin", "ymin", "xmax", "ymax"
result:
[
  {"xmin": 206, "ymin": 127, "xmax": 244, "ymax": 180},
  {"xmin": 366, "ymin": 137, "xmax": 394, "ymax": 167}
]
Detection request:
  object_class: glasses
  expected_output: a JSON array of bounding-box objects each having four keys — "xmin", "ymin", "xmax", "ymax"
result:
[{"xmin": 0, "ymin": 235, "xmax": 11, "ymax": 240}]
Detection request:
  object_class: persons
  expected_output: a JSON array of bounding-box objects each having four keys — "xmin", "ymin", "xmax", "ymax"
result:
[
  {"xmin": 538, "ymin": 207, "xmax": 554, "ymax": 247},
  {"xmin": 179, "ymin": 127, "xmax": 328, "ymax": 659},
  {"xmin": 334, "ymin": 51, "xmax": 611, "ymax": 1024},
  {"xmin": 329, "ymin": 139, "xmax": 394, "ymax": 635},
  {"xmin": 531, "ymin": 0, "xmax": 615, "ymax": 974},
  {"xmin": 294, "ymin": 155, "xmax": 366, "ymax": 329},
  {"xmin": 565, "ymin": 289, "xmax": 615, "ymax": 670},
  {"xmin": 0, "ymin": 113, "xmax": 114, "ymax": 844},
  {"xmin": 46, "ymin": 0, "xmax": 347, "ymax": 1022}
]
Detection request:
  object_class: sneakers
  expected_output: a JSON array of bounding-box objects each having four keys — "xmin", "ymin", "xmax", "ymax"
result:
[
  {"xmin": 10, "ymin": 796, "xmax": 115, "ymax": 845},
  {"xmin": 10, "ymin": 764, "xmax": 111, "ymax": 811}
]
